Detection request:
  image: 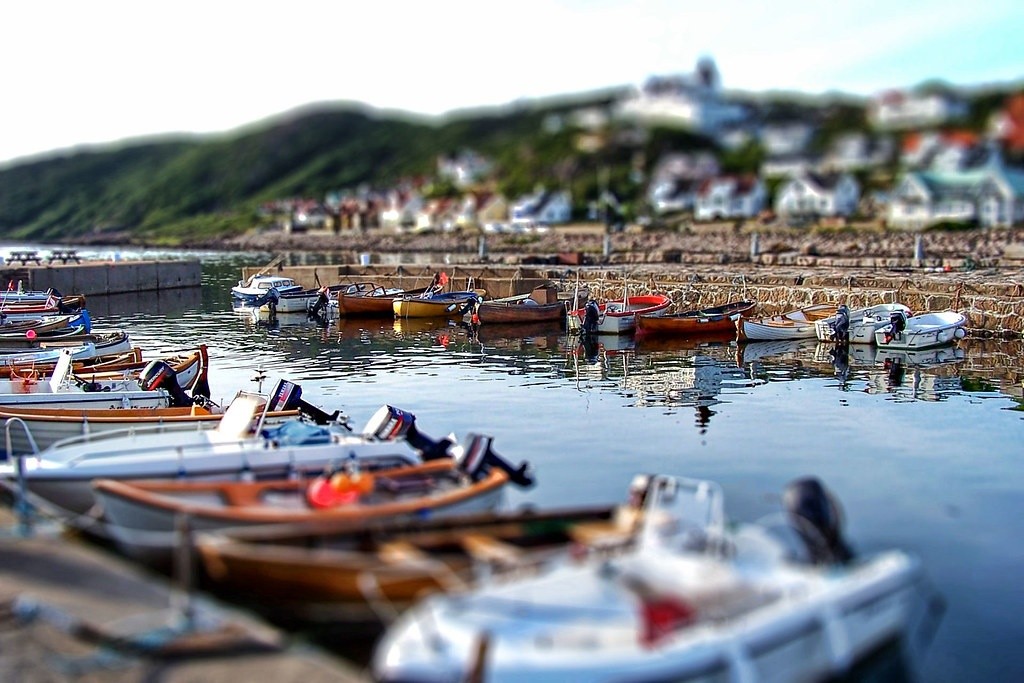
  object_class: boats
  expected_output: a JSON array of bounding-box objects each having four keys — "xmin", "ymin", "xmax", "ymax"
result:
[{"xmin": 2, "ymin": 271, "xmax": 968, "ymax": 683}]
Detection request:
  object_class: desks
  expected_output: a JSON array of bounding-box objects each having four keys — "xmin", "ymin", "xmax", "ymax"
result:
[
  {"xmin": 7, "ymin": 251, "xmax": 41, "ymax": 266},
  {"xmin": 47, "ymin": 249, "xmax": 83, "ymax": 266}
]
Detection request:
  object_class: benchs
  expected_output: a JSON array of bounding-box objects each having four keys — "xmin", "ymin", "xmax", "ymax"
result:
[
  {"xmin": 914, "ymin": 324, "xmax": 941, "ymax": 329},
  {"xmin": 463, "ymin": 532, "xmax": 526, "ymax": 570},
  {"xmin": 810, "ymin": 312, "xmax": 837, "ymax": 319},
  {"xmin": 790, "ymin": 319, "xmax": 816, "ymax": 325},
  {"xmin": 379, "ymin": 540, "xmax": 466, "ymax": 594},
  {"xmin": 6, "ymin": 258, "xmax": 41, "ymax": 261},
  {"xmin": 47, "ymin": 256, "xmax": 83, "ymax": 260}
]
